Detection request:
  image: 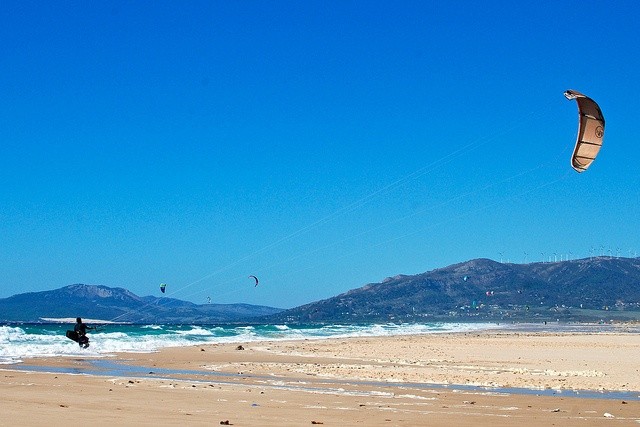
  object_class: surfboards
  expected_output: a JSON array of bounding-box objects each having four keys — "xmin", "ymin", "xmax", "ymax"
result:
[{"xmin": 65, "ymin": 330, "xmax": 89, "ymax": 347}]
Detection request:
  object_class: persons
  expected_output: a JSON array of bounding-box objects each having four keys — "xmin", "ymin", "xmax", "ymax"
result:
[{"xmin": 74, "ymin": 318, "xmax": 95, "ymax": 348}]
